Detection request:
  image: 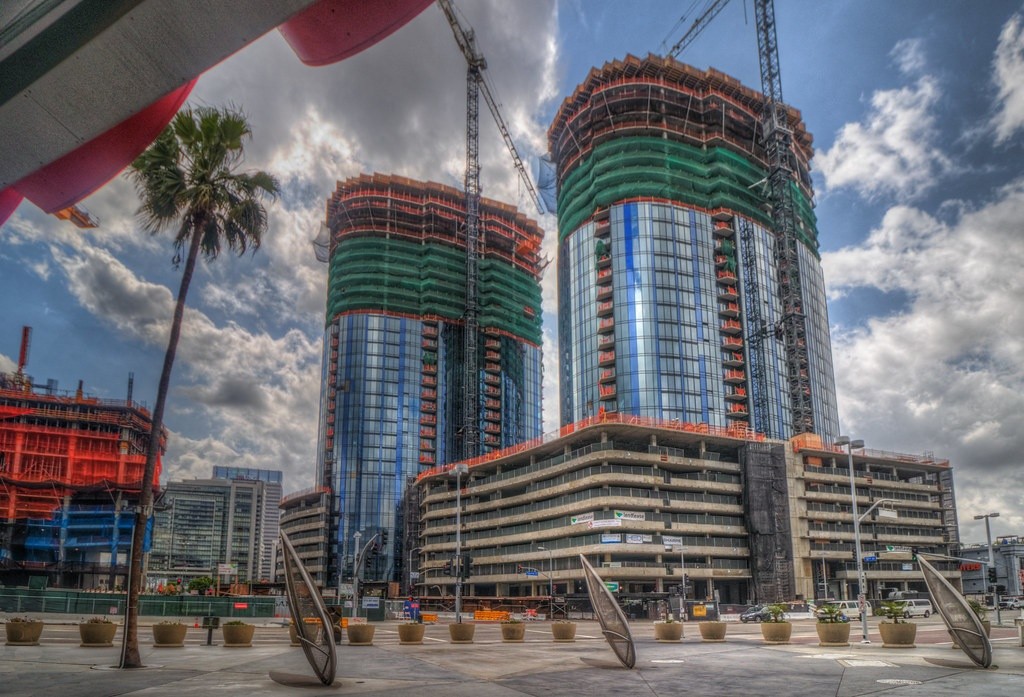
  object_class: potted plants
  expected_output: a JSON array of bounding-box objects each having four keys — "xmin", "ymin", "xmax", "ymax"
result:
[
  {"xmin": 655, "ymin": 620, "xmax": 683, "ymax": 643},
  {"xmin": 760, "ymin": 606, "xmax": 791, "ymax": 644},
  {"xmin": 347, "ymin": 623, "xmax": 376, "ymax": 646},
  {"xmin": 876, "ymin": 600, "xmax": 916, "ymax": 648},
  {"xmin": 950, "ymin": 600, "xmax": 990, "ymax": 649},
  {"xmin": 816, "ymin": 605, "xmax": 850, "ymax": 647},
  {"xmin": 223, "ymin": 620, "xmax": 255, "ymax": 647},
  {"xmin": 501, "ymin": 618, "xmax": 525, "ymax": 642},
  {"xmin": 79, "ymin": 615, "xmax": 117, "ymax": 648},
  {"xmin": 5, "ymin": 615, "xmax": 44, "ymax": 646},
  {"xmin": 398, "ymin": 620, "xmax": 425, "ymax": 645},
  {"xmin": 152, "ymin": 620, "xmax": 187, "ymax": 648},
  {"xmin": 551, "ymin": 621, "xmax": 577, "ymax": 642},
  {"xmin": 605, "ymin": 621, "xmax": 628, "ymax": 642},
  {"xmin": 699, "ymin": 619, "xmax": 727, "ymax": 642}
]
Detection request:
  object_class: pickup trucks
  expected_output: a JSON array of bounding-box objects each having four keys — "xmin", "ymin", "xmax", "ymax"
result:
[{"xmin": 998, "ymin": 598, "xmax": 1024, "ymax": 610}]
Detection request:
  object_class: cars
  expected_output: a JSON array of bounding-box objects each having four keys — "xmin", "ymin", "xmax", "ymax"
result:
[{"xmin": 740, "ymin": 607, "xmax": 785, "ymax": 622}]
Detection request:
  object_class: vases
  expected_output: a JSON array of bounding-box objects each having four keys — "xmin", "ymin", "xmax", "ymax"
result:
[
  {"xmin": 449, "ymin": 623, "xmax": 475, "ymax": 643},
  {"xmin": 290, "ymin": 622, "xmax": 321, "ymax": 647}
]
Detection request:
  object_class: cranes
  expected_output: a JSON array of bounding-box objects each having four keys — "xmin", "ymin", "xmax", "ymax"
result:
[
  {"xmin": 436, "ymin": 0, "xmax": 549, "ymax": 456},
  {"xmin": 646, "ymin": 1, "xmax": 818, "ymax": 435}
]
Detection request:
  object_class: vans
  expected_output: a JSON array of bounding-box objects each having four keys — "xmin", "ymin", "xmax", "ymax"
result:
[
  {"xmin": 883, "ymin": 599, "xmax": 933, "ymax": 619},
  {"xmin": 815, "ymin": 601, "xmax": 873, "ymax": 623}
]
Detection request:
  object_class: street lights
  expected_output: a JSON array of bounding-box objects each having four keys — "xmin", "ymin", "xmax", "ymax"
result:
[
  {"xmin": 833, "ymin": 435, "xmax": 872, "ymax": 644},
  {"xmin": 973, "ymin": 512, "xmax": 1002, "ymax": 625},
  {"xmin": 664, "ymin": 544, "xmax": 687, "ymax": 600},
  {"xmin": 353, "ymin": 532, "xmax": 362, "ymax": 616},
  {"xmin": 537, "ymin": 547, "xmax": 552, "ymax": 619},
  {"xmin": 449, "ymin": 464, "xmax": 469, "ymax": 624}
]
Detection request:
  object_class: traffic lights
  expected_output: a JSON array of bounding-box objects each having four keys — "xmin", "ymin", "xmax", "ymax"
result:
[
  {"xmin": 1001, "ymin": 585, "xmax": 1006, "ymax": 592},
  {"xmin": 518, "ymin": 565, "xmax": 522, "ymax": 574},
  {"xmin": 987, "ymin": 567, "xmax": 997, "ymax": 583},
  {"xmin": 911, "ymin": 549, "xmax": 918, "ymax": 560},
  {"xmin": 465, "ymin": 553, "xmax": 475, "ymax": 578},
  {"xmin": 851, "ymin": 548, "xmax": 857, "ymax": 561},
  {"xmin": 444, "ymin": 560, "xmax": 453, "ymax": 577}
]
